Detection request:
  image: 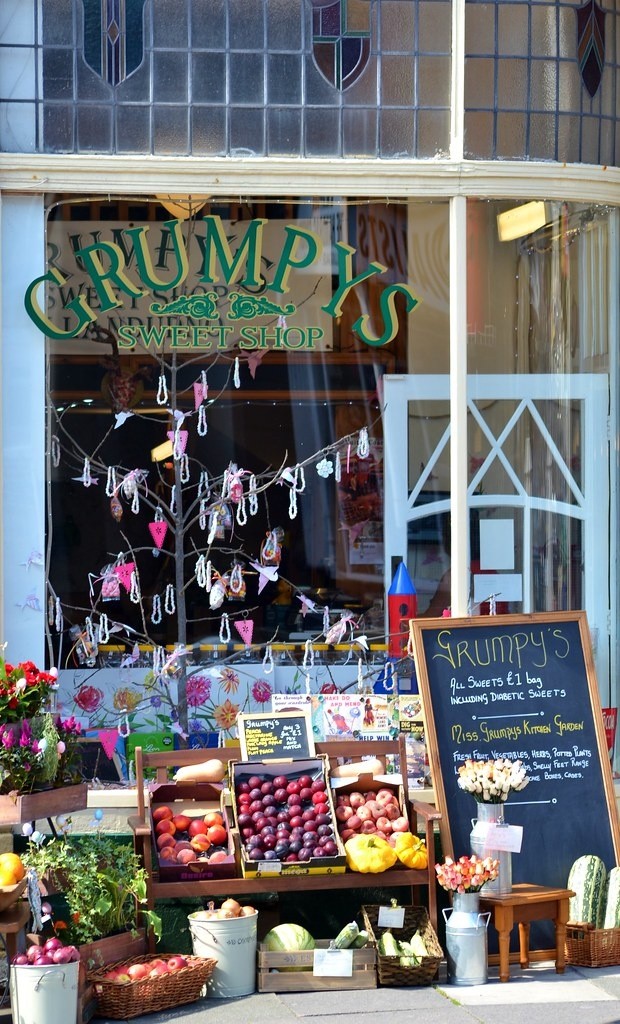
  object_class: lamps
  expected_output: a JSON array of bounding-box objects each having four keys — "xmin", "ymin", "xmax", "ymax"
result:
[{"xmin": 497, "ymin": 200, "xmax": 554, "ymax": 241}]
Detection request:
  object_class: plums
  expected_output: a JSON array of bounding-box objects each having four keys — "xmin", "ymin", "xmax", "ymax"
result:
[{"xmin": 237, "ymin": 776, "xmax": 337, "ymax": 862}]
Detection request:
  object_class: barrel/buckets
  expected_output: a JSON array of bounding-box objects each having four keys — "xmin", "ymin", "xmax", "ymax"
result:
[
  {"xmin": 10, "ymin": 960, "xmax": 80, "ymax": 1024},
  {"xmin": 187, "ymin": 910, "xmax": 259, "ymax": 998}
]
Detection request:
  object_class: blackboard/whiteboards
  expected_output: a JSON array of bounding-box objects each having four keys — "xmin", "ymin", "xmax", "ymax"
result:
[
  {"xmin": 238, "ymin": 711, "xmax": 317, "ymax": 763},
  {"xmin": 76, "ymin": 738, "xmax": 124, "ymax": 783},
  {"xmin": 408, "ymin": 609, "xmax": 620, "ymax": 968}
]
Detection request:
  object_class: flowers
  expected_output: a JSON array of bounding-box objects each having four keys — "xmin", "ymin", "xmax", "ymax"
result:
[
  {"xmin": 18, "ymin": 810, "xmax": 106, "ymax": 873},
  {"xmin": 434, "ymin": 855, "xmax": 501, "ymax": 894},
  {"xmin": 0, "ymin": 641, "xmax": 89, "ymax": 796}
]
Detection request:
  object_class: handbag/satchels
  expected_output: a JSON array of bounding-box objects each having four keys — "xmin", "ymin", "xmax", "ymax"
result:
[{"xmin": 363, "ymin": 719, "xmax": 369, "ymax": 727}]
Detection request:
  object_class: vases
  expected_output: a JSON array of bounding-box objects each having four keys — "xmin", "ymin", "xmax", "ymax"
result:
[
  {"xmin": 442, "ymin": 892, "xmax": 491, "ymax": 985},
  {"xmin": 469, "ymin": 804, "xmax": 512, "ymax": 895}
]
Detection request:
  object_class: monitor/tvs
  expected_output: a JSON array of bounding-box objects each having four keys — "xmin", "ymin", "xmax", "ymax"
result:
[{"xmin": 407, "ymin": 491, "xmax": 452, "ymax": 543}]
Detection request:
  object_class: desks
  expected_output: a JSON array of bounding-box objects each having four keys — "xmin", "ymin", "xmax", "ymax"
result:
[
  {"xmin": 448, "ymin": 884, "xmax": 576, "ymax": 981},
  {"xmin": 0, "ymin": 902, "xmax": 31, "ymax": 969}
]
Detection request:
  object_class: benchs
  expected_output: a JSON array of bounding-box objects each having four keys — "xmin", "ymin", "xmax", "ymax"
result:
[{"xmin": 127, "ymin": 731, "xmax": 444, "ymax": 954}]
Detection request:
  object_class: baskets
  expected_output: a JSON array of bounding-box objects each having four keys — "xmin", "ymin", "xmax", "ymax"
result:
[
  {"xmin": 86, "ymin": 954, "xmax": 219, "ymax": 1019},
  {"xmin": 361, "ymin": 904, "xmax": 445, "ymax": 985},
  {"xmin": 564, "ymin": 921, "xmax": 620, "ymax": 967}
]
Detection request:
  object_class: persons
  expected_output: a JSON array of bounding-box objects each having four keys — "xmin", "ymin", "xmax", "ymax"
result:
[{"xmin": 416, "ymin": 508, "xmax": 511, "ymax": 617}]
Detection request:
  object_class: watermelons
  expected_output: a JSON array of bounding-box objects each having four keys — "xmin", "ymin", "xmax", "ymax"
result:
[{"xmin": 263, "ymin": 923, "xmax": 317, "ymax": 973}]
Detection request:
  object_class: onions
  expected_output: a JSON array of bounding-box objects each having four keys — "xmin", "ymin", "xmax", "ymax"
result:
[
  {"xmin": 9, "ymin": 938, "xmax": 80, "ymax": 965},
  {"xmin": 192, "ymin": 898, "xmax": 255, "ymax": 920}
]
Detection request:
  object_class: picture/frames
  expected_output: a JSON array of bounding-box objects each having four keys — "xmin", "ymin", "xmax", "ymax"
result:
[
  {"xmin": 408, "ymin": 491, "xmax": 452, "ymax": 542},
  {"xmin": 456, "ymin": 756, "xmax": 530, "ymax": 804}
]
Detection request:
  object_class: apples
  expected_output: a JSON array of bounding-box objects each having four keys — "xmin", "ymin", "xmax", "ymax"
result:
[{"xmin": 98, "ymin": 957, "xmax": 203, "ymax": 983}]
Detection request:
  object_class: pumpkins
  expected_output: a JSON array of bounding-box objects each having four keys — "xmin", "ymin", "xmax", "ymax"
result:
[
  {"xmin": 173, "ymin": 758, "xmax": 224, "ymax": 783},
  {"xmin": 330, "ymin": 759, "xmax": 384, "ymax": 777},
  {"xmin": 394, "ymin": 832, "xmax": 428, "ymax": 870},
  {"xmin": 343, "ymin": 833, "xmax": 398, "ymax": 873},
  {"xmin": 567, "ymin": 854, "xmax": 620, "ymax": 930},
  {"xmin": 0, "ymin": 853, "xmax": 24, "ymax": 887}
]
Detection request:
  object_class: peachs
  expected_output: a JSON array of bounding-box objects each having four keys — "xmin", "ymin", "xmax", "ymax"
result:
[
  {"xmin": 151, "ymin": 806, "xmax": 228, "ymax": 865},
  {"xmin": 336, "ymin": 788, "xmax": 409, "ymax": 848}
]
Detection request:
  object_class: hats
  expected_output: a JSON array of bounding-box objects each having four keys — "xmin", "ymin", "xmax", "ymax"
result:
[
  {"xmin": 328, "ymin": 710, "xmax": 331, "ymax": 713},
  {"xmin": 366, "ymin": 699, "xmax": 370, "ymax": 703}
]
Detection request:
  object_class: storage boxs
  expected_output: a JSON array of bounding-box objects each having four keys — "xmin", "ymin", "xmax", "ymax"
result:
[
  {"xmin": 147, "ymin": 781, "xmax": 235, "ymax": 883},
  {"xmin": 22, "ymin": 852, "xmax": 116, "ymax": 899},
  {"xmin": 0, "ymin": 782, "xmax": 88, "ymax": 827},
  {"xmin": 75, "ymin": 926, "xmax": 146, "ymax": 1024},
  {"xmin": 224, "ymin": 752, "xmax": 346, "ymax": 878},
  {"xmin": 256, "ymin": 939, "xmax": 378, "ymax": 993},
  {"xmin": 329, "ymin": 774, "xmax": 413, "ymax": 873}
]
